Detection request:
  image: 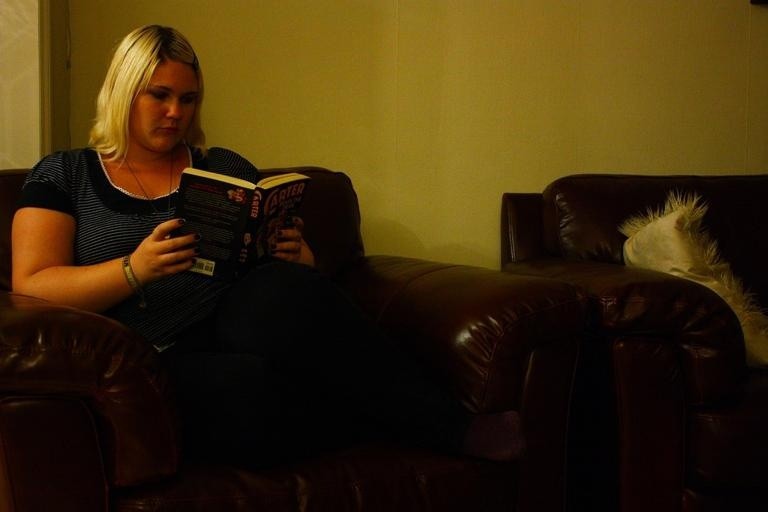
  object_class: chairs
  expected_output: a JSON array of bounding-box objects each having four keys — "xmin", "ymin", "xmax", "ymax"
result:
[
  {"xmin": 501, "ymin": 176, "xmax": 768, "ymax": 508},
  {"xmin": 0, "ymin": 170, "xmax": 615, "ymax": 512}
]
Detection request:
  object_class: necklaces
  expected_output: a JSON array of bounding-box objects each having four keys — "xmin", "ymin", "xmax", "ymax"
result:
[{"xmin": 123, "ymin": 148, "xmax": 175, "ymax": 239}]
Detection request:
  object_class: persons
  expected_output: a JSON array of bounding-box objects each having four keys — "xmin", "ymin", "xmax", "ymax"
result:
[{"xmin": 9, "ymin": 26, "xmax": 462, "ymax": 472}]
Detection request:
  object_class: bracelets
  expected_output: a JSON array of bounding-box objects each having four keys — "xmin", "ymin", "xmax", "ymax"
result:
[{"xmin": 122, "ymin": 254, "xmax": 147, "ymax": 310}]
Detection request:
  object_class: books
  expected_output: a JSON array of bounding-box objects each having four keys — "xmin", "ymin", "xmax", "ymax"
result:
[{"xmin": 173, "ymin": 166, "xmax": 312, "ymax": 283}]
